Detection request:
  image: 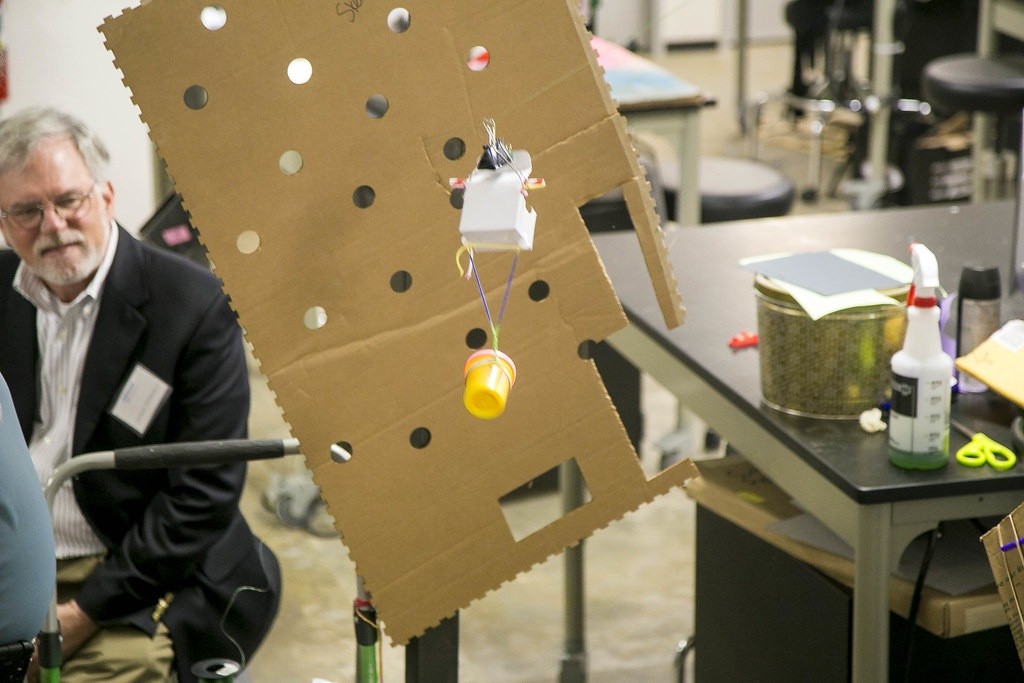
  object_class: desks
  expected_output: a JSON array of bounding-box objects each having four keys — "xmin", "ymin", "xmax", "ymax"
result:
[
  {"xmin": 592, "ymin": 35, "xmax": 717, "ymax": 224},
  {"xmin": 560, "ymin": 200, "xmax": 1024, "ymax": 683}
]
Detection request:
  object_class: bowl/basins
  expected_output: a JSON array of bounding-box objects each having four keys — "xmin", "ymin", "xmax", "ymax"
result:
[{"xmin": 751, "ymin": 269, "xmax": 909, "ymax": 421}]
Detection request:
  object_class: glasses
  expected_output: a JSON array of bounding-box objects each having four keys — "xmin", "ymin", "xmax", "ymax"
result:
[{"xmin": 1, "ymin": 179, "xmax": 98, "ymax": 231}]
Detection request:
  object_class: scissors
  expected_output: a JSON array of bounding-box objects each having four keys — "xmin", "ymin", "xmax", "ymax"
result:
[{"xmin": 950, "ymin": 419, "xmax": 1017, "ymax": 472}]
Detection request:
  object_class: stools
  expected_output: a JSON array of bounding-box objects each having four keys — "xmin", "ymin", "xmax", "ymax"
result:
[{"xmin": 925, "ymin": 55, "xmax": 1024, "ymax": 200}]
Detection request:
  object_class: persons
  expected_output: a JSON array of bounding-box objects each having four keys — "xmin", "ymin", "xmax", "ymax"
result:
[{"xmin": 0, "ymin": 102, "xmax": 277, "ymax": 683}]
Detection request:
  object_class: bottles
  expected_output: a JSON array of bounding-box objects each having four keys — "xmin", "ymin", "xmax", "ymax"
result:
[{"xmin": 956, "ymin": 261, "xmax": 1002, "ymax": 394}]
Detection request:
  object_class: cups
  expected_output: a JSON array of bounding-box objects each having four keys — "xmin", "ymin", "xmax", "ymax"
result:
[{"xmin": 462, "ymin": 350, "xmax": 517, "ymax": 419}]
